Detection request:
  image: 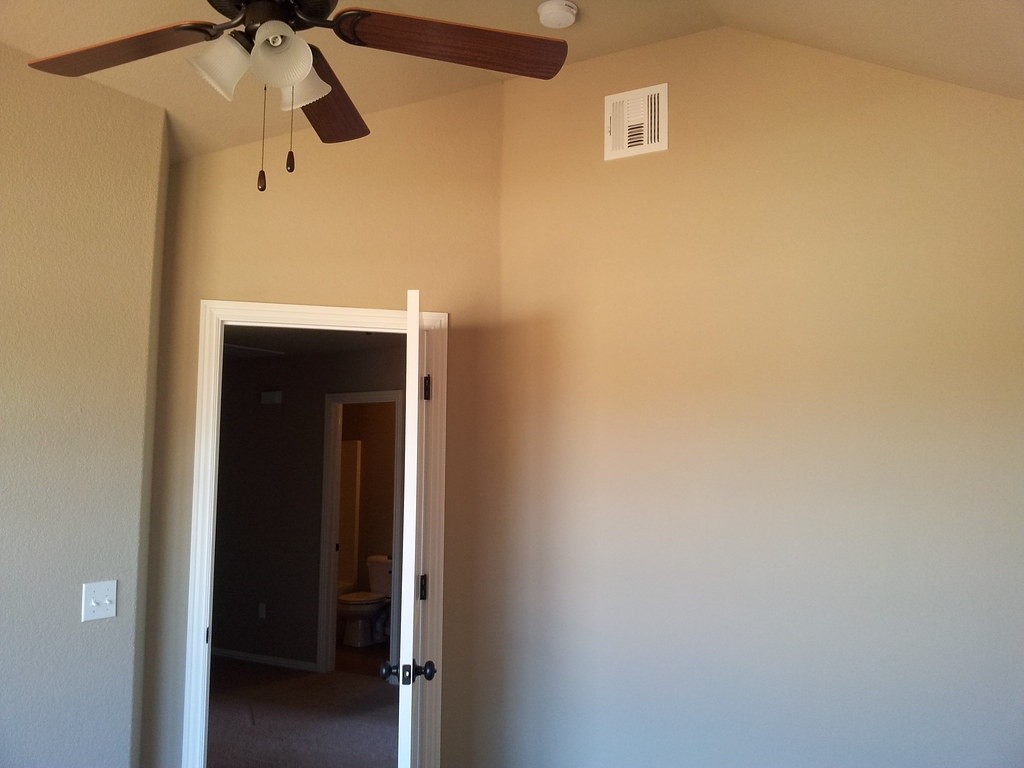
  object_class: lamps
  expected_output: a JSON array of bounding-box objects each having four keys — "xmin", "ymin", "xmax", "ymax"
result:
[
  {"xmin": 279, "ymin": 66, "xmax": 333, "ymax": 114},
  {"xmin": 186, "ymin": 21, "xmax": 255, "ymax": 103},
  {"xmin": 249, "ymin": 15, "xmax": 314, "ymax": 89}
]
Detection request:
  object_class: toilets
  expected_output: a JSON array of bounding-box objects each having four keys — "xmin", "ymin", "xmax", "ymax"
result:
[{"xmin": 336, "ymin": 554, "xmax": 395, "ymax": 649}]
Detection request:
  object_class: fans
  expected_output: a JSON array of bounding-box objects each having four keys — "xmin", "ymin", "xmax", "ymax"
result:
[{"xmin": 26, "ymin": 1, "xmax": 569, "ymax": 192}]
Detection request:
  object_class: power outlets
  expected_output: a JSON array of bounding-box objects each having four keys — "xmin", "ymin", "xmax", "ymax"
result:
[{"xmin": 81, "ymin": 579, "xmax": 118, "ymax": 623}]
